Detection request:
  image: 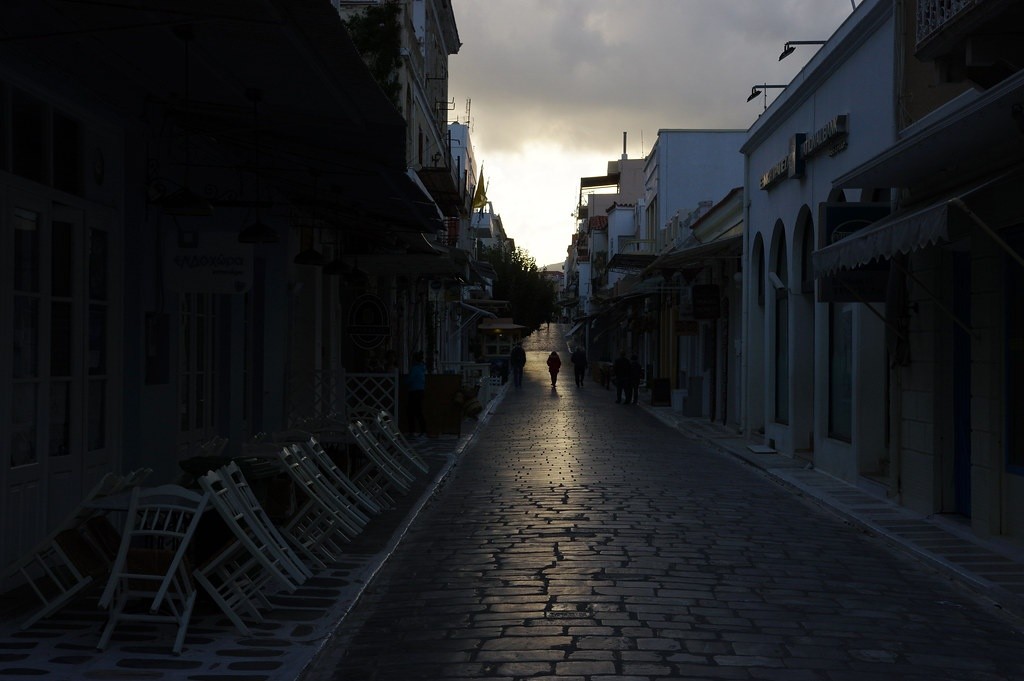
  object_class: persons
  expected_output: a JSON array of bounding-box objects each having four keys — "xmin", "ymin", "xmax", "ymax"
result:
[
  {"xmin": 572, "ymin": 345, "xmax": 589, "ymax": 387},
  {"xmin": 547, "ymin": 351, "xmax": 561, "ymax": 387},
  {"xmin": 614, "ymin": 351, "xmax": 641, "ymax": 405},
  {"xmin": 600, "ymin": 352, "xmax": 612, "ymax": 391},
  {"xmin": 384, "ymin": 350, "xmax": 400, "ymax": 377},
  {"xmin": 512, "ymin": 342, "xmax": 526, "ymax": 388},
  {"xmin": 404, "ymin": 351, "xmax": 427, "ymax": 440}
]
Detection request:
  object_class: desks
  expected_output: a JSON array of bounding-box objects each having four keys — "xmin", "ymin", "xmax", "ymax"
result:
[
  {"xmin": 317, "ymin": 428, "xmax": 365, "ymax": 472},
  {"xmin": 224, "ymin": 445, "xmax": 296, "ymax": 512},
  {"xmin": 83, "ymin": 487, "xmax": 220, "ymax": 571}
]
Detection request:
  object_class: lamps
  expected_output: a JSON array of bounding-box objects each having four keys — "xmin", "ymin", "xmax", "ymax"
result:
[
  {"xmin": 239, "ymin": 87, "xmax": 281, "ymax": 242},
  {"xmin": 779, "ymin": 41, "xmax": 827, "ymax": 61},
  {"xmin": 341, "ymin": 195, "xmax": 374, "ymax": 281},
  {"xmin": 747, "ymin": 85, "xmax": 788, "ymax": 102},
  {"xmin": 146, "ymin": 22, "xmax": 214, "ymax": 215},
  {"xmin": 294, "ymin": 169, "xmax": 328, "ymax": 265}
]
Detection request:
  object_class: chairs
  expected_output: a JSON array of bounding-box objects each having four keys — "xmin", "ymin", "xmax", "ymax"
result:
[{"xmin": 0, "ymin": 408, "xmax": 429, "ymax": 656}]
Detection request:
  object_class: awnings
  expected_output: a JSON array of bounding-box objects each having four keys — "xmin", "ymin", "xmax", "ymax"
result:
[
  {"xmin": 812, "ymin": 160, "xmax": 1023, "ymax": 279},
  {"xmin": 452, "ymin": 301, "xmax": 496, "ymax": 337}
]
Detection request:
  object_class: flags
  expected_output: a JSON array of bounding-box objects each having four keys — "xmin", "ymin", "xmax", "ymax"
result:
[{"xmin": 471, "ymin": 171, "xmax": 487, "ymax": 208}]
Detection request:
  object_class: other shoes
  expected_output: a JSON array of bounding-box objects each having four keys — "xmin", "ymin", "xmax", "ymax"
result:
[
  {"xmin": 614, "ymin": 399, "xmax": 621, "ymax": 404},
  {"xmin": 407, "ymin": 432, "xmax": 416, "ymax": 440},
  {"xmin": 623, "ymin": 399, "xmax": 631, "ymax": 404},
  {"xmin": 417, "ymin": 433, "xmax": 427, "ymax": 439},
  {"xmin": 632, "ymin": 400, "xmax": 636, "ymax": 405}
]
